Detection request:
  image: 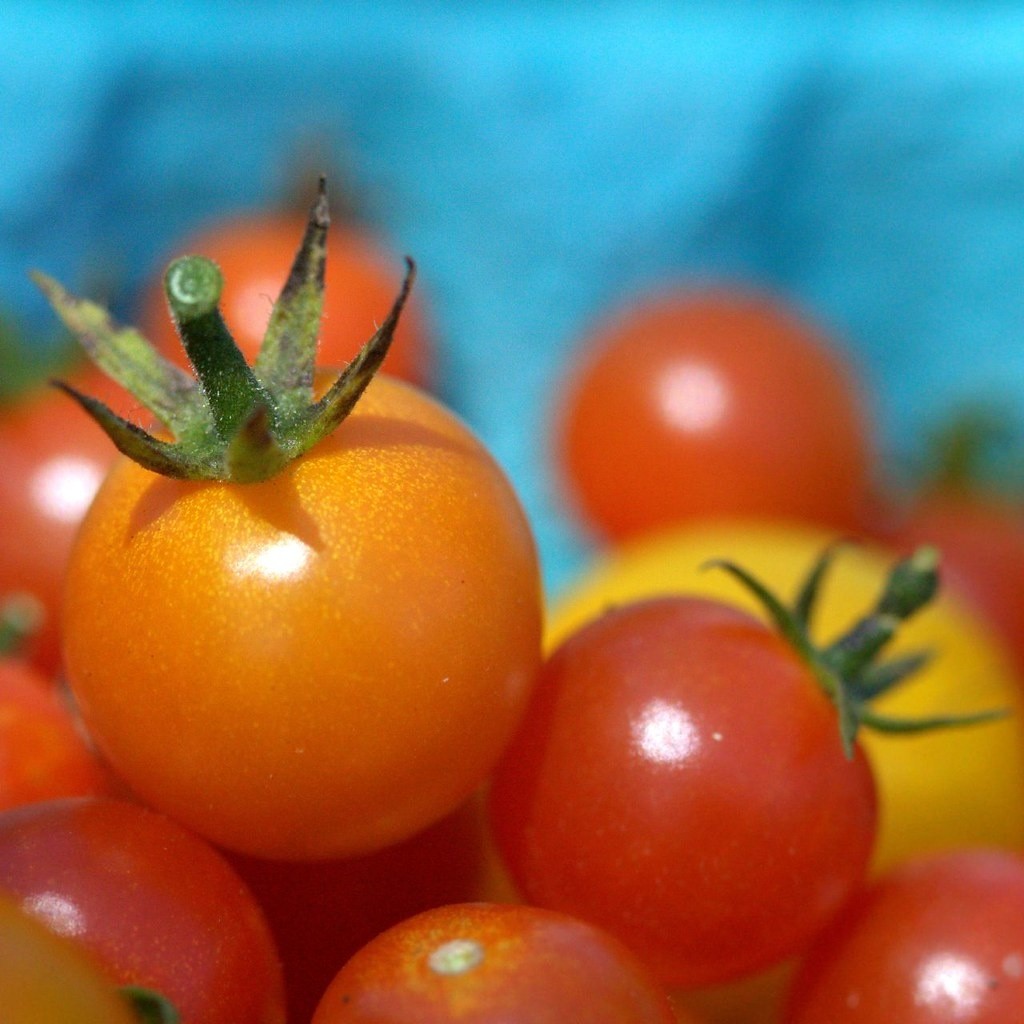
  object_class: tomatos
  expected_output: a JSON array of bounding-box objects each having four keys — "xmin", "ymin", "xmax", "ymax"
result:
[{"xmin": 1, "ymin": 229, "xmax": 1023, "ymax": 1024}]
